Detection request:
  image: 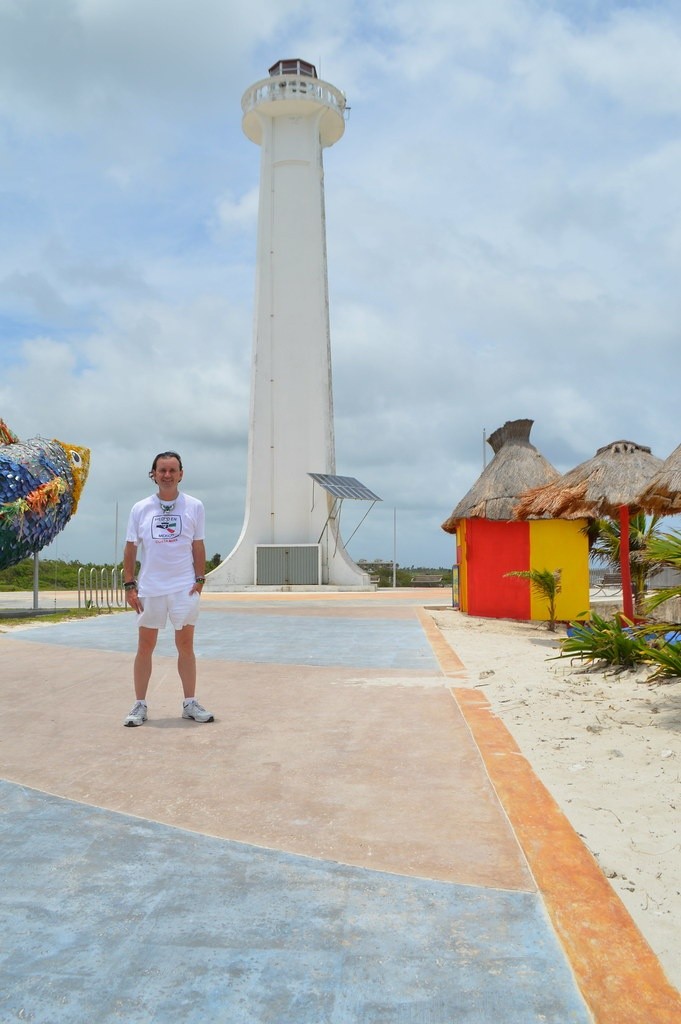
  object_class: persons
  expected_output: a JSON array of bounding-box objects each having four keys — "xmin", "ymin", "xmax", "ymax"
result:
[{"xmin": 121, "ymin": 452, "xmax": 215, "ymax": 727}]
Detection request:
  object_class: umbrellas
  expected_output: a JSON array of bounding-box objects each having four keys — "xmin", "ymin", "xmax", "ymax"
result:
[
  {"xmin": 513, "ymin": 439, "xmax": 671, "ymax": 631},
  {"xmin": 638, "ymin": 431, "xmax": 681, "ymax": 519}
]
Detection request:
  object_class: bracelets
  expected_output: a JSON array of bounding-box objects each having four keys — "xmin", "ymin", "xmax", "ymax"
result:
[
  {"xmin": 123, "ymin": 580, "xmax": 137, "ymax": 590},
  {"xmin": 195, "ymin": 576, "xmax": 206, "ymax": 584}
]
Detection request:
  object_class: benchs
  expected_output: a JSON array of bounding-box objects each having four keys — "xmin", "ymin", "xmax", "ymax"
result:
[
  {"xmin": 370, "ymin": 575, "xmax": 380, "ymax": 584},
  {"xmin": 591, "ymin": 572, "xmax": 623, "ymax": 597},
  {"xmin": 411, "ymin": 575, "xmax": 443, "ymax": 587}
]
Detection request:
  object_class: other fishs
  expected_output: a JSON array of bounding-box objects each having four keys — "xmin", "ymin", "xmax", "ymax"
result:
[{"xmin": 0, "ymin": 418, "xmax": 90, "ymax": 575}]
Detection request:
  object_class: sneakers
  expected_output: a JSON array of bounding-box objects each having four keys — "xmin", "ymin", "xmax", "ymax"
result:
[
  {"xmin": 123, "ymin": 701, "xmax": 148, "ymax": 727},
  {"xmin": 183, "ymin": 699, "xmax": 214, "ymax": 723}
]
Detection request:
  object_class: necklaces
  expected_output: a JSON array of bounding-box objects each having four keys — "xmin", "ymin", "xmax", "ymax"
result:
[{"xmin": 158, "ymin": 489, "xmax": 181, "ymax": 512}]
